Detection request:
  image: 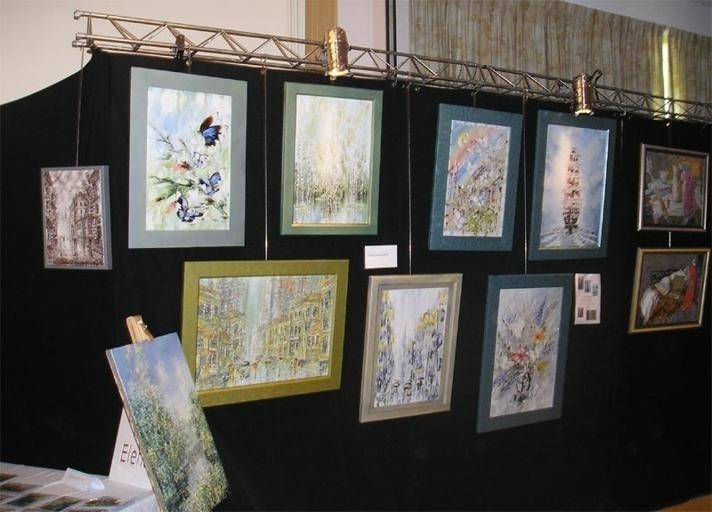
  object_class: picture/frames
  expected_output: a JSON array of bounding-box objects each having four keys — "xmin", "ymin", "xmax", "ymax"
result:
[
  {"xmin": 127, "ymin": 65, "xmax": 248, "ymax": 250},
  {"xmin": 476, "ymin": 271, "xmax": 576, "ymax": 434},
  {"xmin": 180, "ymin": 257, "xmax": 350, "ymax": 407},
  {"xmin": 527, "ymin": 107, "xmax": 616, "ymax": 260},
  {"xmin": 637, "ymin": 140, "xmax": 707, "ymax": 232},
  {"xmin": 428, "ymin": 103, "xmax": 524, "ymax": 253},
  {"xmin": 359, "ymin": 273, "xmax": 464, "ymax": 423},
  {"xmin": 628, "ymin": 248, "xmax": 708, "ymax": 332},
  {"xmin": 281, "ymin": 81, "xmax": 384, "ymax": 237}
]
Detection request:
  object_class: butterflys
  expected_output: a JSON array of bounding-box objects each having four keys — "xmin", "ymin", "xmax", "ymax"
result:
[
  {"xmin": 196, "ymin": 116, "xmax": 223, "ymax": 147},
  {"xmin": 175, "ymin": 195, "xmax": 203, "ymax": 223},
  {"xmin": 191, "ymin": 151, "xmax": 208, "ymax": 167},
  {"xmin": 198, "ymin": 171, "xmax": 221, "ymax": 195}
]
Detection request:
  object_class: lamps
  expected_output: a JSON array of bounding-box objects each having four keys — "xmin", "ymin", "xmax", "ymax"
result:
[{"xmin": 324, "ymin": 25, "xmax": 352, "ymax": 79}]
